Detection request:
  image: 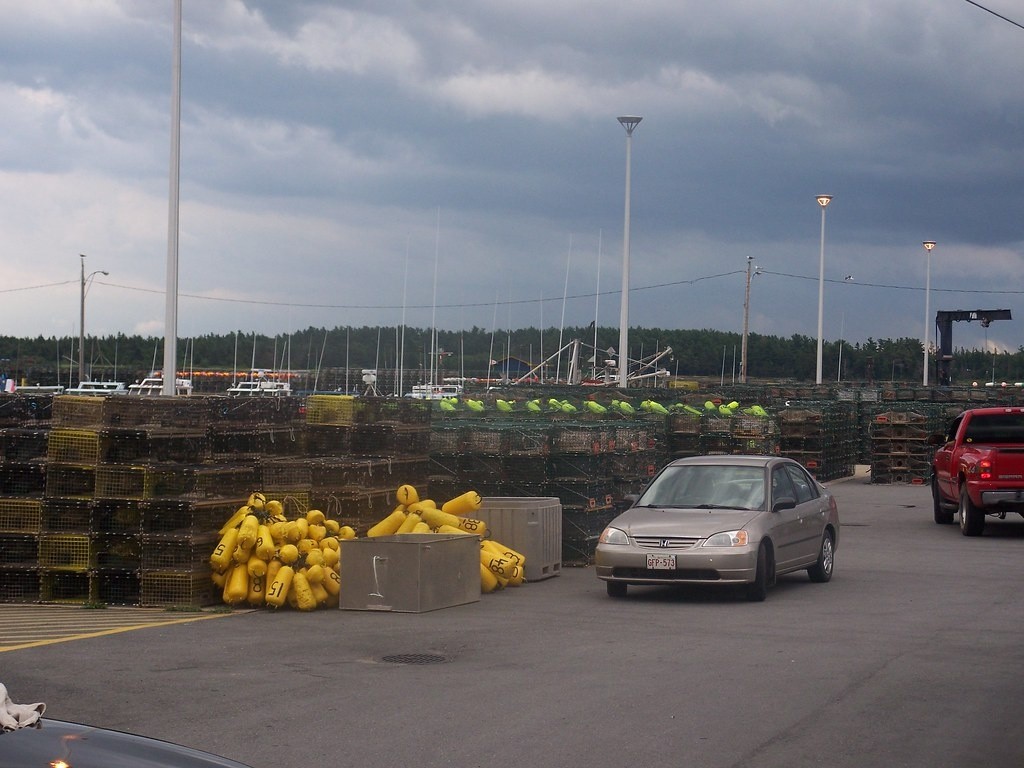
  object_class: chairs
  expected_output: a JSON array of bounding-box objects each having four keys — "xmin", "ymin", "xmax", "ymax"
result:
[
  {"xmin": 746, "ymin": 480, "xmax": 774, "ymax": 510},
  {"xmin": 678, "ymin": 476, "xmax": 716, "ymax": 506}
]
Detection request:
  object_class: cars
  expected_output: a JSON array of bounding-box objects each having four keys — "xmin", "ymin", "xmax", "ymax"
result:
[{"xmin": 594, "ymin": 456, "xmax": 840, "ymax": 602}]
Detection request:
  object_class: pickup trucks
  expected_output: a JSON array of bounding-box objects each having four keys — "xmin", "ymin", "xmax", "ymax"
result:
[{"xmin": 928, "ymin": 408, "xmax": 1024, "ymax": 536}]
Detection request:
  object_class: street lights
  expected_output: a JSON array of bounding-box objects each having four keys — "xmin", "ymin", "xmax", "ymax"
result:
[
  {"xmin": 740, "ymin": 255, "xmax": 762, "ymax": 384},
  {"xmin": 616, "ymin": 116, "xmax": 643, "ymax": 387},
  {"xmin": 813, "ymin": 195, "xmax": 834, "ymax": 384},
  {"xmin": 922, "ymin": 241, "xmax": 937, "ymax": 386},
  {"xmin": 78, "ymin": 254, "xmax": 109, "ymax": 381}
]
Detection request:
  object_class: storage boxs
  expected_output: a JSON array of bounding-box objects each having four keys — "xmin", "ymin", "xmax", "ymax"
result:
[{"xmin": 339, "ymin": 532, "xmax": 481, "ymax": 614}]
